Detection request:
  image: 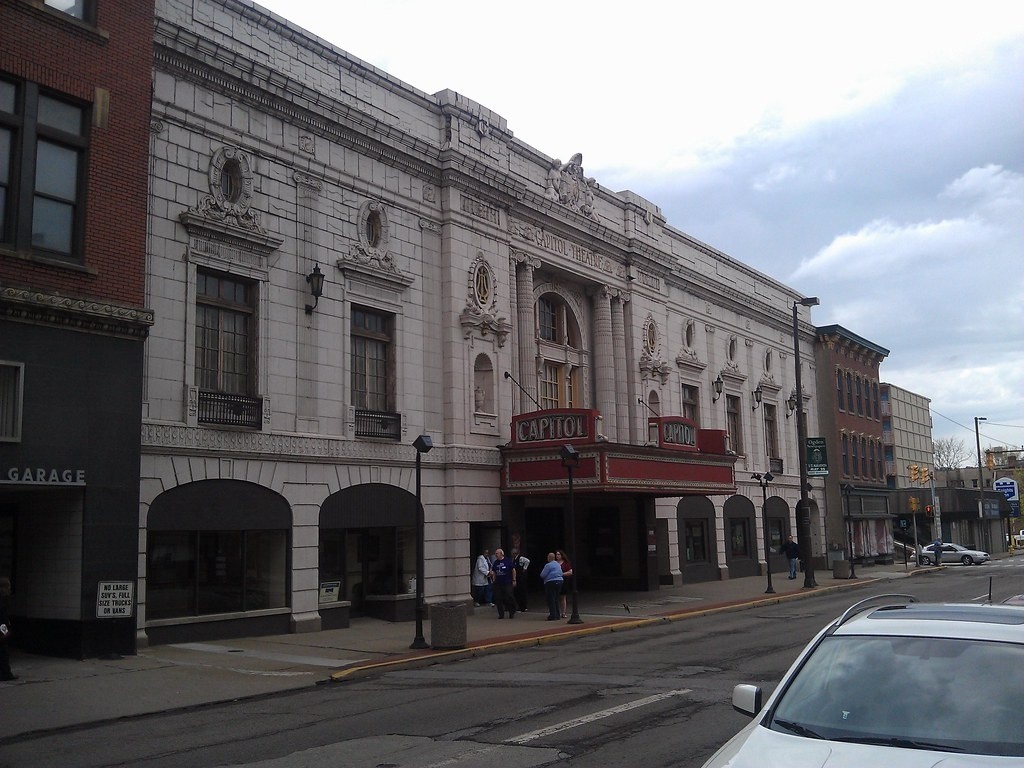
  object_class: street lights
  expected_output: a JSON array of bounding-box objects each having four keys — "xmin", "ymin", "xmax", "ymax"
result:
[
  {"xmin": 560, "ymin": 442, "xmax": 586, "ymax": 625},
  {"xmin": 843, "ymin": 482, "xmax": 859, "ymax": 580},
  {"xmin": 749, "ymin": 472, "xmax": 774, "ymax": 595},
  {"xmin": 975, "ymin": 416, "xmax": 987, "ymax": 550},
  {"xmin": 411, "ymin": 434, "xmax": 434, "ymax": 648},
  {"xmin": 792, "ymin": 297, "xmax": 821, "ymax": 590}
]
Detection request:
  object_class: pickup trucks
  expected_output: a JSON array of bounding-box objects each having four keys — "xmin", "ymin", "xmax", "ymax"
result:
[{"xmin": 1006, "ymin": 529, "xmax": 1024, "ymax": 546}]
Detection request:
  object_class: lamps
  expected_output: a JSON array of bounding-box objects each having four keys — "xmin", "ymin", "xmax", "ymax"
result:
[
  {"xmin": 752, "ymin": 386, "xmax": 762, "ymax": 411},
  {"xmin": 786, "ymin": 396, "xmax": 795, "ymax": 420},
  {"xmin": 596, "ymin": 434, "xmax": 609, "ymax": 442},
  {"xmin": 712, "ymin": 374, "xmax": 723, "ymax": 403},
  {"xmin": 305, "ymin": 263, "xmax": 326, "ymax": 314},
  {"xmin": 727, "ymin": 449, "xmax": 736, "ymax": 455}
]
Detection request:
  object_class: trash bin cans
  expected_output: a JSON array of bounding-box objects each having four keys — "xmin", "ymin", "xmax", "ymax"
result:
[
  {"xmin": 833, "ymin": 560, "xmax": 848, "ymax": 578},
  {"xmin": 431, "ymin": 602, "xmax": 467, "ymax": 647}
]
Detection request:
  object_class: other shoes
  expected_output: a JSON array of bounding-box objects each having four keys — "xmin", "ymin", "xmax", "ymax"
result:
[
  {"xmin": 545, "ymin": 616, "xmax": 560, "ymax": 621},
  {"xmin": 474, "ymin": 602, "xmax": 480, "ymax": 606},
  {"xmin": 488, "ymin": 603, "xmax": 495, "ymax": 607}
]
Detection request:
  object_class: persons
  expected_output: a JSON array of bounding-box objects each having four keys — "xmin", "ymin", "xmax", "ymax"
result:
[
  {"xmin": 0, "ymin": 578, "xmax": 18, "ymax": 681},
  {"xmin": 470, "ymin": 532, "xmax": 573, "ymax": 621},
  {"xmin": 933, "ymin": 538, "xmax": 943, "ymax": 567},
  {"xmin": 778, "ymin": 535, "xmax": 800, "ymax": 579}
]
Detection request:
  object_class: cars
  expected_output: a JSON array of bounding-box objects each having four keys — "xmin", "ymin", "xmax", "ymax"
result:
[
  {"xmin": 909, "ymin": 541, "xmax": 991, "ymax": 567},
  {"xmin": 700, "ymin": 594, "xmax": 1024, "ymax": 767}
]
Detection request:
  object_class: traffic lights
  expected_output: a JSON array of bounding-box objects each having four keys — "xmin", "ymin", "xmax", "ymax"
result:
[
  {"xmin": 921, "ymin": 467, "xmax": 929, "ymax": 486},
  {"xmin": 910, "ymin": 465, "xmax": 919, "ymax": 483},
  {"xmin": 926, "ymin": 505, "xmax": 932, "ymax": 517}
]
